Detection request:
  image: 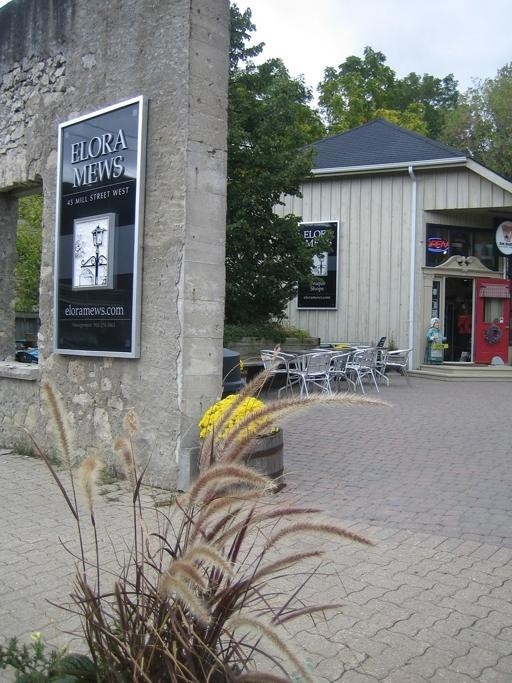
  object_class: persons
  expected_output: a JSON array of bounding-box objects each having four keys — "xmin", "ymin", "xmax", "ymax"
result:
[{"xmin": 424, "ymin": 317, "xmax": 445, "ymax": 365}]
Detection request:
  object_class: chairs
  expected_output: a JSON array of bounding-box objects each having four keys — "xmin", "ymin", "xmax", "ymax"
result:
[{"xmin": 255, "ymin": 333, "xmax": 415, "ymax": 400}]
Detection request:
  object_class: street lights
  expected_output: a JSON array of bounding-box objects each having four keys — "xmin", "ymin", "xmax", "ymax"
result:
[
  {"xmin": 81, "ymin": 224, "xmax": 109, "ymax": 287},
  {"xmin": 318, "ymin": 254, "xmax": 325, "ymax": 276}
]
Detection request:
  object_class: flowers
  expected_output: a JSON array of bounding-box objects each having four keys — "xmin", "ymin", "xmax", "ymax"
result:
[{"xmin": 198, "ymin": 388, "xmax": 281, "ymax": 448}]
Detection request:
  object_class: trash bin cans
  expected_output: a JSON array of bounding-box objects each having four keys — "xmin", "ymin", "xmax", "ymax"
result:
[{"xmin": 223, "ymin": 348, "xmax": 242, "ymax": 399}]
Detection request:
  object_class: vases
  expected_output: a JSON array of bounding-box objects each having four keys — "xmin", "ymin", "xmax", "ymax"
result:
[{"xmin": 206, "ymin": 423, "xmax": 288, "ymax": 499}]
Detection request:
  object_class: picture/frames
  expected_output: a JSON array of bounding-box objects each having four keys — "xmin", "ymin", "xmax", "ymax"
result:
[
  {"xmin": 52, "ymin": 92, "xmax": 148, "ymax": 360},
  {"xmin": 296, "ymin": 219, "xmax": 339, "ymax": 311}
]
